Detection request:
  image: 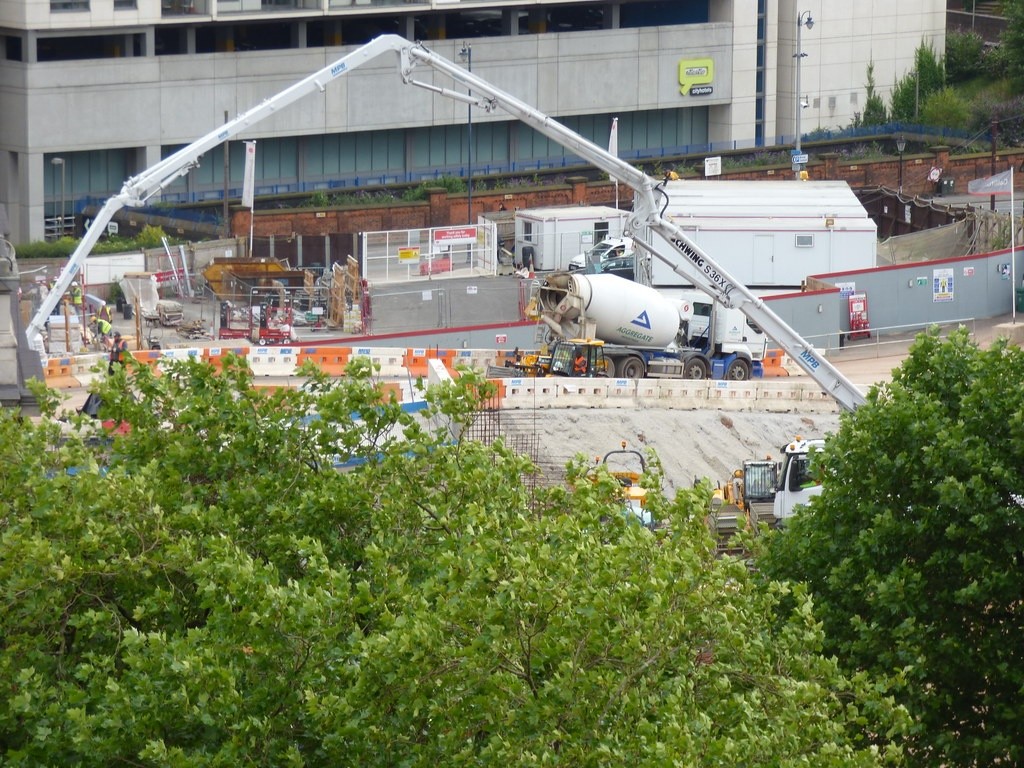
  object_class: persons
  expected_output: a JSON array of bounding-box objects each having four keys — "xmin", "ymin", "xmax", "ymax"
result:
[
  {"xmin": 800, "ymin": 471, "xmax": 822, "ymax": 488},
  {"xmin": 49, "ymin": 276, "xmax": 60, "ymax": 315},
  {"xmin": 499, "ymin": 203, "xmax": 507, "ymax": 211},
  {"xmin": 99, "ymin": 301, "xmax": 112, "ymax": 323},
  {"xmin": 108, "ymin": 332, "xmax": 127, "ymax": 376},
  {"xmin": 70, "ymin": 282, "xmax": 82, "ymax": 313},
  {"xmin": 666, "ymin": 170, "xmax": 679, "ymax": 180},
  {"xmin": 573, "ymin": 350, "xmax": 586, "ymax": 376},
  {"xmin": 90, "ymin": 316, "xmax": 112, "ymax": 338}
]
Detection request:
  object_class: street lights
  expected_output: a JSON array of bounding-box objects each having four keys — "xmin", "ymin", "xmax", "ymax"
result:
[
  {"xmin": 459, "ymin": 40, "xmax": 472, "ymax": 225},
  {"xmin": 51, "ymin": 158, "xmax": 66, "ymax": 237},
  {"xmin": 897, "ymin": 136, "xmax": 907, "ymax": 194},
  {"xmin": 791, "ymin": 10, "xmax": 814, "ymax": 181}
]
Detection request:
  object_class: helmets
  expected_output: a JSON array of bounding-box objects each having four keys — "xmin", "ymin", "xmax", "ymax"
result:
[
  {"xmin": 71, "ymin": 281, "xmax": 77, "ymax": 286},
  {"xmin": 53, "ymin": 276, "xmax": 58, "ymax": 281},
  {"xmin": 90, "ymin": 316, "xmax": 96, "ymax": 322},
  {"xmin": 113, "ymin": 331, "xmax": 120, "ymax": 336}
]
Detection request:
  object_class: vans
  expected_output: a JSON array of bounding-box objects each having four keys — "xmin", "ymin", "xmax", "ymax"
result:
[{"xmin": 568, "ymin": 237, "xmax": 636, "ymax": 271}]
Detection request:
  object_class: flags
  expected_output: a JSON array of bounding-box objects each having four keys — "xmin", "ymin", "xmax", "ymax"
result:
[
  {"xmin": 242, "ymin": 141, "xmax": 256, "ymax": 208},
  {"xmin": 967, "ymin": 170, "xmax": 1011, "ymax": 196},
  {"xmin": 609, "ymin": 120, "xmax": 618, "ymax": 181}
]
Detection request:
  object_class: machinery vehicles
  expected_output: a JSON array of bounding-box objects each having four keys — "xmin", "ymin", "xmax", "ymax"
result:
[
  {"xmin": 704, "ymin": 454, "xmax": 778, "ymax": 572},
  {"xmin": 26, "ymin": 34, "xmax": 872, "ymax": 519},
  {"xmin": 486, "ymin": 338, "xmax": 610, "ymax": 378},
  {"xmin": 568, "ymin": 441, "xmax": 673, "ymax": 546},
  {"xmin": 539, "ymin": 273, "xmax": 769, "ymax": 380}
]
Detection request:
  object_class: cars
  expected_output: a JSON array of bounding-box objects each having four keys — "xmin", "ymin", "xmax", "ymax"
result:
[{"xmin": 573, "ymin": 256, "xmax": 634, "ymax": 281}]
